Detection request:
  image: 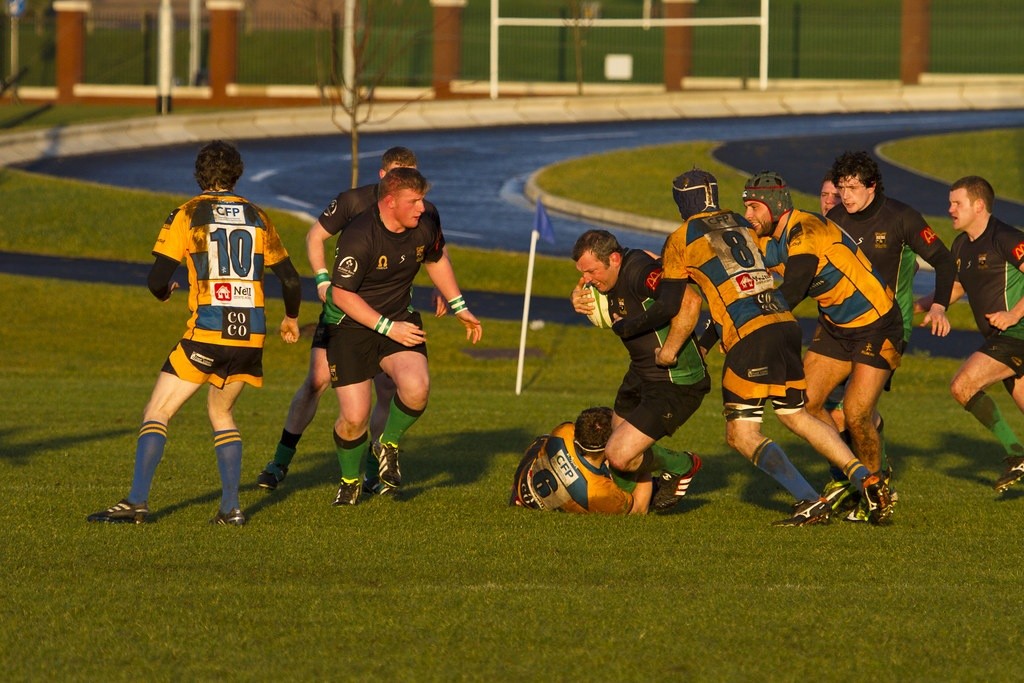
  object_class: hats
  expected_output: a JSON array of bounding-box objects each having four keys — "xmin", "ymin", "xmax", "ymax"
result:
[
  {"xmin": 742, "ymin": 172, "xmax": 793, "ymax": 224},
  {"xmin": 671, "ymin": 170, "xmax": 718, "ymax": 218}
]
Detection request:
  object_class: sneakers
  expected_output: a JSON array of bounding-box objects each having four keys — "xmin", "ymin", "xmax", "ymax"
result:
[
  {"xmin": 209, "ymin": 507, "xmax": 246, "ymax": 527},
  {"xmin": 363, "ymin": 475, "xmax": 379, "ymax": 497},
  {"xmin": 371, "ymin": 432, "xmax": 401, "ymax": 488},
  {"xmin": 652, "ymin": 451, "xmax": 700, "ymax": 513},
  {"xmin": 861, "ymin": 474, "xmax": 895, "ymax": 524},
  {"xmin": 993, "ymin": 453, "xmax": 1024, "ymax": 490},
  {"xmin": 88, "ymin": 495, "xmax": 149, "ymax": 525},
  {"xmin": 820, "ymin": 481, "xmax": 860, "ymax": 514},
  {"xmin": 257, "ymin": 460, "xmax": 288, "ymax": 490},
  {"xmin": 771, "ymin": 499, "xmax": 833, "ymax": 529},
  {"xmin": 882, "ymin": 466, "xmax": 893, "ymax": 485},
  {"xmin": 332, "ymin": 479, "xmax": 362, "ymax": 507},
  {"xmin": 841, "ymin": 493, "xmax": 872, "ymax": 524}
]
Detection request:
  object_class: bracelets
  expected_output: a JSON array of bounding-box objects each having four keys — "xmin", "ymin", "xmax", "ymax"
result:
[
  {"xmin": 314, "ymin": 268, "xmax": 331, "ymax": 289},
  {"xmin": 448, "ymin": 296, "xmax": 469, "ymax": 315},
  {"xmin": 373, "ymin": 316, "xmax": 394, "ymax": 336}
]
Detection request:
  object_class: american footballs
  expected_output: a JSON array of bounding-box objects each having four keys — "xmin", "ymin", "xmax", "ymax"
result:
[{"xmin": 581, "ymin": 282, "xmax": 613, "ymax": 329}]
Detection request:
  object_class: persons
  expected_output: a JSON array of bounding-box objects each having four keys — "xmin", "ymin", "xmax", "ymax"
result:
[
  {"xmin": 508, "ymin": 405, "xmax": 654, "ymax": 516},
  {"xmin": 912, "ymin": 176, "xmax": 1024, "ymax": 493},
  {"xmin": 570, "ymin": 230, "xmax": 712, "ymax": 513},
  {"xmin": 256, "ymin": 143, "xmax": 417, "ymax": 489},
  {"xmin": 86, "ymin": 140, "xmax": 300, "ymax": 525},
  {"xmin": 324, "ymin": 167, "xmax": 483, "ymax": 507},
  {"xmin": 612, "ymin": 149, "xmax": 957, "ymax": 528}
]
